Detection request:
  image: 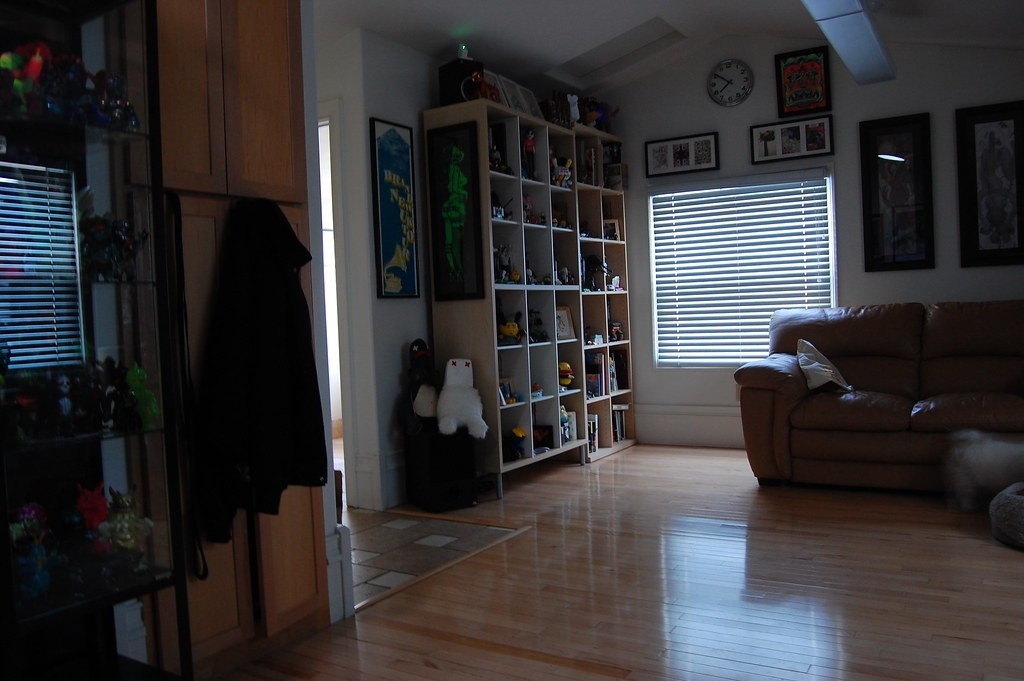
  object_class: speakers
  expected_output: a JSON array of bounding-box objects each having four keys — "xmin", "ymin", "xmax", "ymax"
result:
[{"xmin": 438, "ymin": 58, "xmax": 484, "ymax": 108}]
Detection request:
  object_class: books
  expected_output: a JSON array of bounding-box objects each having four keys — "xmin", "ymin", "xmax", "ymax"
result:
[
  {"xmin": 567, "ymin": 411, "xmax": 578, "ymax": 440},
  {"xmin": 611, "ymin": 404, "xmax": 630, "ymax": 442},
  {"xmin": 609, "ymin": 349, "xmax": 629, "ymax": 392},
  {"xmin": 587, "ymin": 413, "xmax": 599, "ymax": 453},
  {"xmin": 586, "ymin": 352, "xmax": 606, "ymax": 396},
  {"xmin": 498, "ymin": 378, "xmax": 514, "ymax": 406}
]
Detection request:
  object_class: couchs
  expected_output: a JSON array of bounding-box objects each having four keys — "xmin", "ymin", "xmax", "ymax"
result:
[{"xmin": 734, "ymin": 299, "xmax": 1024, "ymax": 494}]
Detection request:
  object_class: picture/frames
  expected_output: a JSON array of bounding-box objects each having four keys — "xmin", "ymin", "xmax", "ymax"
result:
[
  {"xmin": 955, "ymin": 99, "xmax": 1024, "ymax": 269},
  {"xmin": 556, "ymin": 306, "xmax": 575, "ymax": 340},
  {"xmin": 775, "ymin": 46, "xmax": 832, "ymax": 120},
  {"xmin": 427, "ymin": 121, "xmax": 486, "ymax": 302},
  {"xmin": 603, "ymin": 219, "xmax": 620, "ymax": 241},
  {"xmin": 369, "ymin": 117, "xmax": 421, "ymax": 299},
  {"xmin": 645, "ymin": 132, "xmax": 720, "ymax": 178},
  {"xmin": 498, "ymin": 74, "xmax": 546, "ymax": 121},
  {"xmin": 483, "ymin": 69, "xmax": 509, "ymax": 107},
  {"xmin": 859, "ymin": 113, "xmax": 936, "ymax": 273},
  {"xmin": 750, "ymin": 115, "xmax": 835, "ymax": 165}
]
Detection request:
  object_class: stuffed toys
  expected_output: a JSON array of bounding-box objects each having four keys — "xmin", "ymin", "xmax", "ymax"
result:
[{"xmin": 414, "ymin": 358, "xmax": 489, "ymax": 439}]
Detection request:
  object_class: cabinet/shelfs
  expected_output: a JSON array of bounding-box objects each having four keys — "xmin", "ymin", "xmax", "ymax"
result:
[
  {"xmin": 131, "ymin": 191, "xmax": 328, "ymax": 674},
  {"xmin": 0, "ymin": 0, "xmax": 190, "ymax": 681},
  {"xmin": 119, "ymin": 0, "xmax": 308, "ymax": 207},
  {"xmin": 422, "ymin": 99, "xmax": 638, "ymax": 499}
]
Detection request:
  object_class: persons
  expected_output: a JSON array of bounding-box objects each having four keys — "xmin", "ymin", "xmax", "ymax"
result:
[{"xmin": 522, "ymin": 129, "xmax": 541, "ymax": 182}]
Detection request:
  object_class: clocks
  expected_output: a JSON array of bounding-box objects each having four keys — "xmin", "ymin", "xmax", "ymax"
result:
[{"xmin": 706, "ymin": 59, "xmax": 753, "ymax": 107}]
